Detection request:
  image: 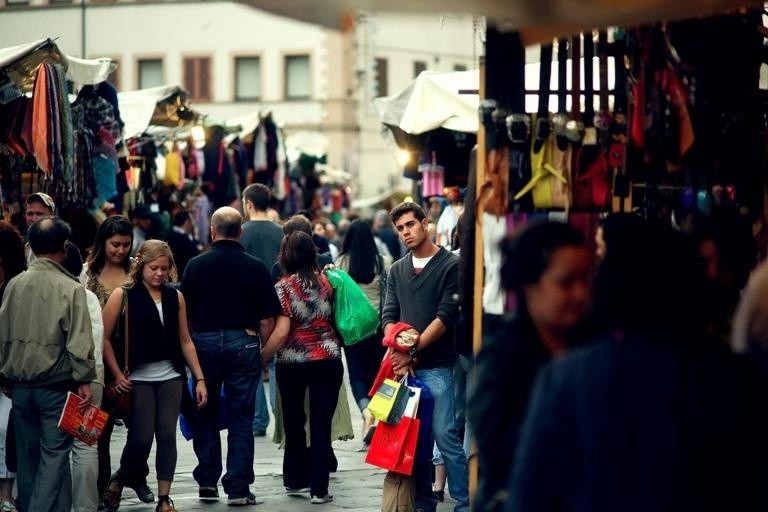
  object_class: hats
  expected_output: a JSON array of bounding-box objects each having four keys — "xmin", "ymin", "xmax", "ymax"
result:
[{"xmin": 26, "ymin": 192, "xmax": 55, "ymax": 212}]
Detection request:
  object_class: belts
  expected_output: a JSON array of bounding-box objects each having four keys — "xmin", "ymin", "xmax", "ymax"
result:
[{"xmin": 480, "ymin": 25, "xmax": 628, "ymax": 157}]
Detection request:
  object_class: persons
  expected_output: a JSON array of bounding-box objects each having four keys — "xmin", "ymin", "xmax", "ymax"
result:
[
  {"xmin": 468, "ymin": 210, "xmax": 768, "ymax": 511},
  {"xmin": 1, "ymin": 183, "xmax": 466, "ymax": 510}
]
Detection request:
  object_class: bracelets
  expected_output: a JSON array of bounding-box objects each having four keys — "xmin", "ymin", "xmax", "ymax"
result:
[{"xmin": 196, "ymin": 379, "xmax": 205, "ymax": 383}]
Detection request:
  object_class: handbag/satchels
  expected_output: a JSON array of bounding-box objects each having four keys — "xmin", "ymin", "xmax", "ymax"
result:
[
  {"xmin": 365, "ymin": 378, "xmax": 421, "ymax": 512},
  {"xmin": 180, "ymin": 377, "xmax": 229, "ymax": 440},
  {"xmin": 101, "ymin": 378, "xmax": 132, "ymax": 419},
  {"xmin": 152, "ymin": 142, "xmax": 205, "ymax": 190},
  {"xmin": 380, "ymin": 274, "xmax": 387, "ymax": 319}
]
